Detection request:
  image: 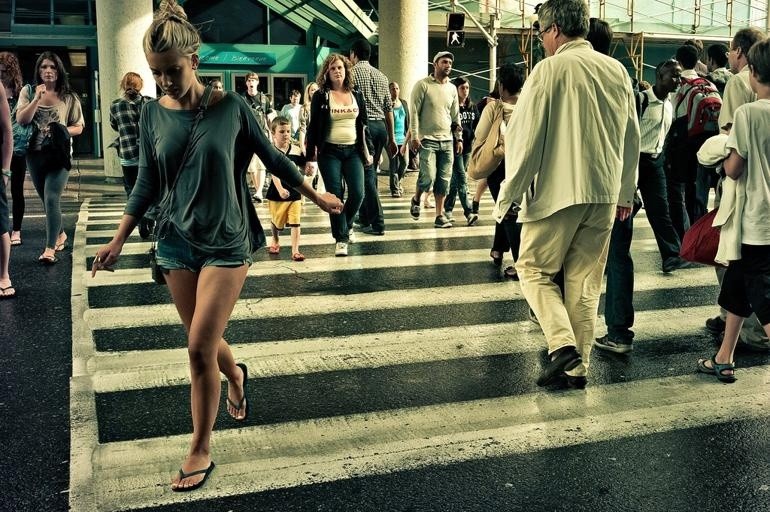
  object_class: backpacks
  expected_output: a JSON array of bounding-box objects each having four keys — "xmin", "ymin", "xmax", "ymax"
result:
[{"xmin": 673, "ymin": 75, "xmax": 723, "ymax": 146}]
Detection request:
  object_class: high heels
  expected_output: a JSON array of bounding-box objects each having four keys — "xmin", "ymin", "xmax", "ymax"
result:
[
  {"xmin": 504, "ymin": 266, "xmax": 520, "ymax": 281},
  {"xmin": 490, "ymin": 248, "xmax": 504, "ymax": 266}
]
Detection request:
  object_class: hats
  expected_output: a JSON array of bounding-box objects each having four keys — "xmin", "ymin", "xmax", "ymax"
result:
[{"xmin": 432, "ymin": 50, "xmax": 455, "ymax": 64}]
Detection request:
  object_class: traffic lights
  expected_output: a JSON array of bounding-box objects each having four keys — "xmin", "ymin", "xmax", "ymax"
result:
[{"xmin": 445, "ymin": 10, "xmax": 467, "ymax": 50}]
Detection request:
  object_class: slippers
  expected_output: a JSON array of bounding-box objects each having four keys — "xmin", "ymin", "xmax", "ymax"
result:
[
  {"xmin": 38, "ymin": 236, "xmax": 68, "ymax": 264},
  {"xmin": 291, "ymin": 252, "xmax": 306, "ymax": 261},
  {"xmin": 0, "ymin": 278, "xmax": 17, "ymax": 299},
  {"xmin": 10, "ymin": 230, "xmax": 23, "ymax": 246},
  {"xmin": 171, "ymin": 460, "xmax": 216, "ymax": 493},
  {"xmin": 269, "ymin": 243, "xmax": 280, "ymax": 255},
  {"xmin": 225, "ymin": 363, "xmax": 249, "ymax": 422}
]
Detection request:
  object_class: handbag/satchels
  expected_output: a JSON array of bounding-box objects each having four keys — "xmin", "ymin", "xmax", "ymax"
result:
[
  {"xmin": 467, "ymin": 98, "xmax": 508, "ymax": 181},
  {"xmin": 678, "ymin": 205, "xmax": 728, "ymax": 269},
  {"xmin": 10, "ymin": 82, "xmax": 34, "ymax": 156},
  {"xmin": 148, "ymin": 246, "xmax": 168, "ymax": 285},
  {"xmin": 360, "ymin": 123, "xmax": 375, "ymax": 167}
]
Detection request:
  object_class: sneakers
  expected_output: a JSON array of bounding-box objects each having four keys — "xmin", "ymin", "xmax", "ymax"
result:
[
  {"xmin": 593, "ymin": 334, "xmax": 634, "ymax": 354},
  {"xmin": 706, "ymin": 315, "xmax": 745, "ymax": 346},
  {"xmin": 433, "ymin": 211, "xmax": 456, "ymax": 228},
  {"xmin": 465, "ymin": 196, "xmax": 480, "ymax": 226},
  {"xmin": 410, "ymin": 196, "xmax": 421, "ymax": 221},
  {"xmin": 352, "ymin": 214, "xmax": 385, "ymax": 236},
  {"xmin": 391, "ymin": 182, "xmax": 404, "ymax": 198},
  {"xmin": 335, "ymin": 228, "xmax": 356, "ymax": 256}
]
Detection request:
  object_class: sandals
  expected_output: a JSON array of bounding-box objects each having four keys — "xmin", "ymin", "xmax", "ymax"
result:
[{"xmin": 696, "ymin": 353, "xmax": 737, "ymax": 384}]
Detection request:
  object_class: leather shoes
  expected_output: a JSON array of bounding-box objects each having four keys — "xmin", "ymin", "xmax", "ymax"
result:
[
  {"xmin": 661, "ymin": 254, "xmax": 693, "ymax": 274},
  {"xmin": 535, "ymin": 346, "xmax": 588, "ymax": 387}
]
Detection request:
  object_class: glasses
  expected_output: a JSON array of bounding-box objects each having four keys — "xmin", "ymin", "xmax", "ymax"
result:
[
  {"xmin": 658, "ymin": 58, "xmax": 679, "ymax": 73},
  {"xmin": 724, "ymin": 47, "xmax": 738, "ymax": 59},
  {"xmin": 536, "ymin": 24, "xmax": 563, "ymax": 42}
]
